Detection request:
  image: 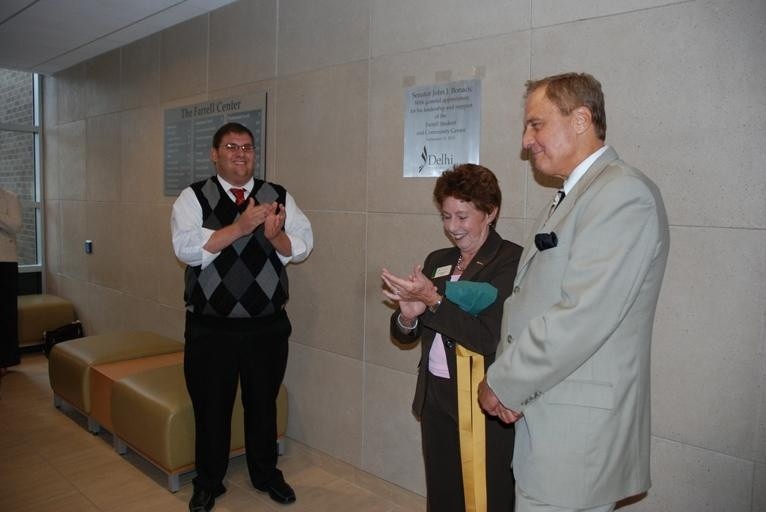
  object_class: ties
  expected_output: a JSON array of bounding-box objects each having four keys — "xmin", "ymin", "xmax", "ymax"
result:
[{"xmin": 229, "ymin": 188, "xmax": 248, "ymax": 204}]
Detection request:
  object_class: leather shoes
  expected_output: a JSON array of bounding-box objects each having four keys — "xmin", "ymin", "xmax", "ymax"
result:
[
  {"xmin": 256, "ymin": 475, "xmax": 296, "ymax": 504},
  {"xmin": 188, "ymin": 490, "xmax": 219, "ymax": 511}
]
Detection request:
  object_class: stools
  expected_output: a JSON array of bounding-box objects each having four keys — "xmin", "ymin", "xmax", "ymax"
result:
[{"xmin": 16, "ymin": 292, "xmax": 73, "ymax": 356}]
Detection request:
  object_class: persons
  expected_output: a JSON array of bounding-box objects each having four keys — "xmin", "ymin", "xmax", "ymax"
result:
[
  {"xmin": 476, "ymin": 70, "xmax": 668, "ymax": 511},
  {"xmin": 170, "ymin": 121, "xmax": 317, "ymax": 510},
  {"xmin": 379, "ymin": 163, "xmax": 525, "ymax": 511},
  {"xmin": 0, "ymin": 184, "xmax": 26, "ymax": 375}
]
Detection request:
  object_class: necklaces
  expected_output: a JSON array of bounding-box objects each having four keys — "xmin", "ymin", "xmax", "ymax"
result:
[{"xmin": 456, "ymin": 255, "xmax": 466, "ymax": 271}]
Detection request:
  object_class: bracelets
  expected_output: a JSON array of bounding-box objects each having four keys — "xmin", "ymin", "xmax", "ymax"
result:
[{"xmin": 397, "ymin": 313, "xmax": 419, "ymax": 329}]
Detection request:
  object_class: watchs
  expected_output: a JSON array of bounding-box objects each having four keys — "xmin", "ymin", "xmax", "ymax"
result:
[{"xmin": 428, "ymin": 294, "xmax": 442, "ymax": 313}]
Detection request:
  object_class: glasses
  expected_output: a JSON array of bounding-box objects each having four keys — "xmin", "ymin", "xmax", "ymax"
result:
[{"xmin": 219, "ymin": 144, "xmax": 255, "ymax": 152}]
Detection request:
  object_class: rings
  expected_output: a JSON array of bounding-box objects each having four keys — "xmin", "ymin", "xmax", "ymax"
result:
[{"xmin": 396, "ymin": 289, "xmax": 400, "ymax": 295}]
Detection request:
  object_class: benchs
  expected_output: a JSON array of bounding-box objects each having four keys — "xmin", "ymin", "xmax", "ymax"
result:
[{"xmin": 48, "ymin": 329, "xmax": 287, "ymax": 494}]
